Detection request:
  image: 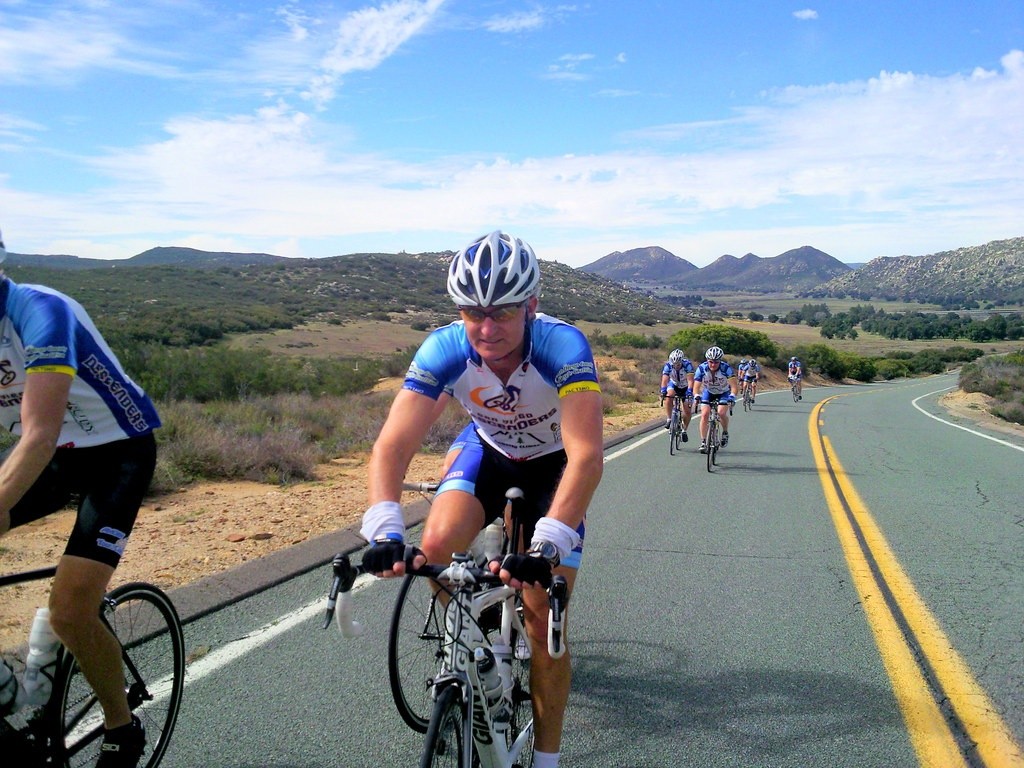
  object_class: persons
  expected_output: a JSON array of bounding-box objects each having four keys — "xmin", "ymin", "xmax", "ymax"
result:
[
  {"xmin": 360, "ymin": 230, "xmax": 603, "ymax": 767},
  {"xmin": 738, "ymin": 359, "xmax": 760, "ymax": 406},
  {"xmin": 692, "ymin": 346, "xmax": 738, "ymax": 452},
  {"xmin": 661, "ymin": 348, "xmax": 694, "ymax": 443},
  {"xmin": 0, "ymin": 229, "xmax": 160, "ymax": 767},
  {"xmin": 787, "ymin": 357, "xmax": 802, "ymax": 400}
]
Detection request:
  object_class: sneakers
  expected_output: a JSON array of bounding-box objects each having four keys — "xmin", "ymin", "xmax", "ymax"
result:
[
  {"xmin": 665, "ymin": 421, "xmax": 670, "ymax": 429},
  {"xmin": 95, "ymin": 714, "xmax": 146, "ymax": 768},
  {"xmin": 682, "ymin": 431, "xmax": 688, "ymax": 442},
  {"xmin": 719, "ymin": 432, "xmax": 729, "ymax": 448},
  {"xmin": 698, "ymin": 443, "xmax": 707, "ymax": 451}
]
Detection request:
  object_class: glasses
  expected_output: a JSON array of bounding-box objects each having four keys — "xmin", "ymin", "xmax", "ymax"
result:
[
  {"xmin": 460, "ymin": 299, "xmax": 528, "ymax": 324},
  {"xmin": 708, "ymin": 360, "xmax": 720, "ymax": 364}
]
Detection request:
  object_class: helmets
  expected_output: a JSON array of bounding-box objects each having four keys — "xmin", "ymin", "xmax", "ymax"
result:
[
  {"xmin": 446, "ymin": 230, "xmax": 539, "ymax": 307},
  {"xmin": 749, "ymin": 359, "xmax": 756, "ymax": 367},
  {"xmin": 791, "ymin": 357, "xmax": 796, "ymax": 361},
  {"xmin": 741, "ymin": 358, "xmax": 747, "ymax": 365},
  {"xmin": 705, "ymin": 346, "xmax": 724, "ymax": 360},
  {"xmin": 669, "ymin": 349, "xmax": 684, "ymax": 365}
]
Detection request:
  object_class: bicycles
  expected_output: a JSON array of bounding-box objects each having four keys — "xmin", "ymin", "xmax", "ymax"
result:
[
  {"xmin": 317, "ymin": 483, "xmax": 570, "ymax": 768},
  {"xmin": 0, "ymin": 490, "xmax": 188, "ymax": 768},
  {"xmin": 693, "ymin": 395, "xmax": 735, "ymax": 472},
  {"xmin": 660, "ymin": 390, "xmax": 692, "ymax": 456},
  {"xmin": 787, "ymin": 376, "xmax": 801, "ymax": 403},
  {"xmin": 737, "ymin": 378, "xmax": 758, "ymax": 412}
]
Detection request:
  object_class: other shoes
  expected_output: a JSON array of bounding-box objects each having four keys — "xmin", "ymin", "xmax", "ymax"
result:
[
  {"xmin": 799, "ymin": 396, "xmax": 802, "ymax": 400},
  {"xmin": 751, "ymin": 400, "xmax": 754, "ymax": 403}
]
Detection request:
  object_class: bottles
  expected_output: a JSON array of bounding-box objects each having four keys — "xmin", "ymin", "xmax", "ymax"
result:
[
  {"xmin": 490, "ymin": 634, "xmax": 512, "ymax": 722},
  {"xmin": 21, "ymin": 606, "xmax": 61, "ymax": 708},
  {"xmin": 474, "ymin": 645, "xmax": 504, "ymax": 712},
  {"xmin": 0, "ymin": 655, "xmax": 25, "ymax": 717}
]
